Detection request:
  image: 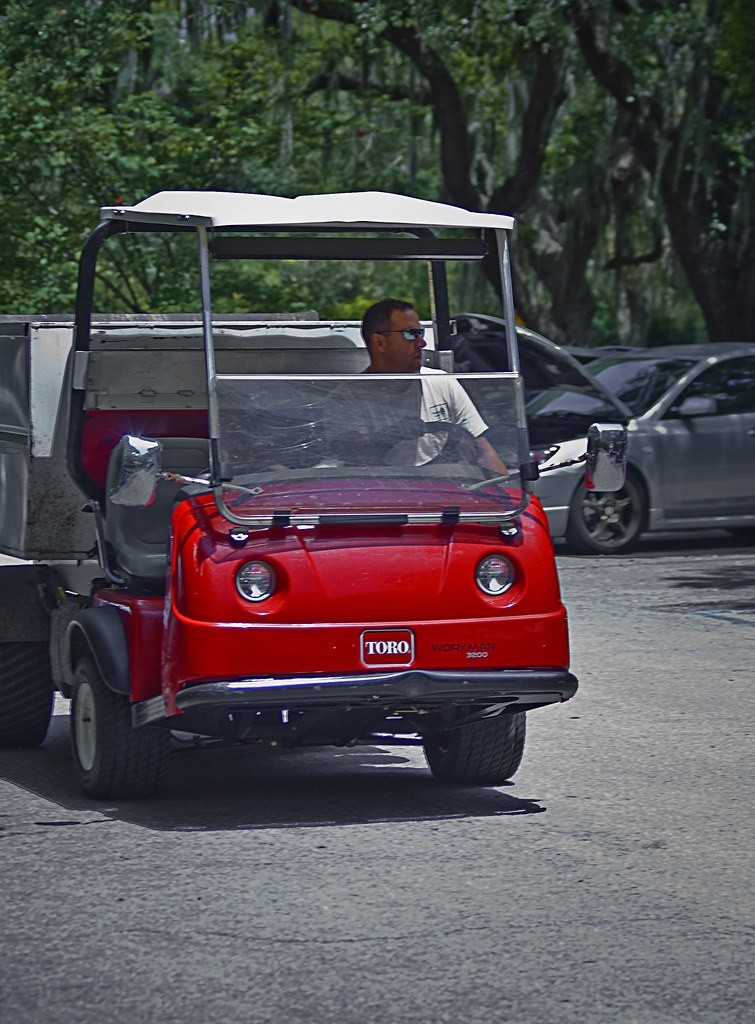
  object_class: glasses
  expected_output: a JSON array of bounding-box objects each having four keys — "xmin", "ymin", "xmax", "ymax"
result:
[{"xmin": 377, "ymin": 328, "xmax": 424, "ymax": 340}]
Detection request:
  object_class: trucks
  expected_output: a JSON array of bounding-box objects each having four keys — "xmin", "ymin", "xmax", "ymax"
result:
[{"xmin": 0, "ymin": 188, "xmax": 578, "ymax": 801}]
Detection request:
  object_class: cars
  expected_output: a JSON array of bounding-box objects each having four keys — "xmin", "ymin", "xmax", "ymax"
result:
[{"xmin": 449, "ymin": 310, "xmax": 755, "ymax": 556}]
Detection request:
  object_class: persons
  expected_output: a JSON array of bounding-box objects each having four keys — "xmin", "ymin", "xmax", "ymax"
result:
[{"xmin": 322, "ymin": 299, "xmax": 509, "ymax": 484}]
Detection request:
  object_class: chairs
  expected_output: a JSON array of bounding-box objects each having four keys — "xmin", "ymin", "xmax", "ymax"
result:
[{"xmin": 103, "ymin": 438, "xmax": 212, "ymax": 589}]
